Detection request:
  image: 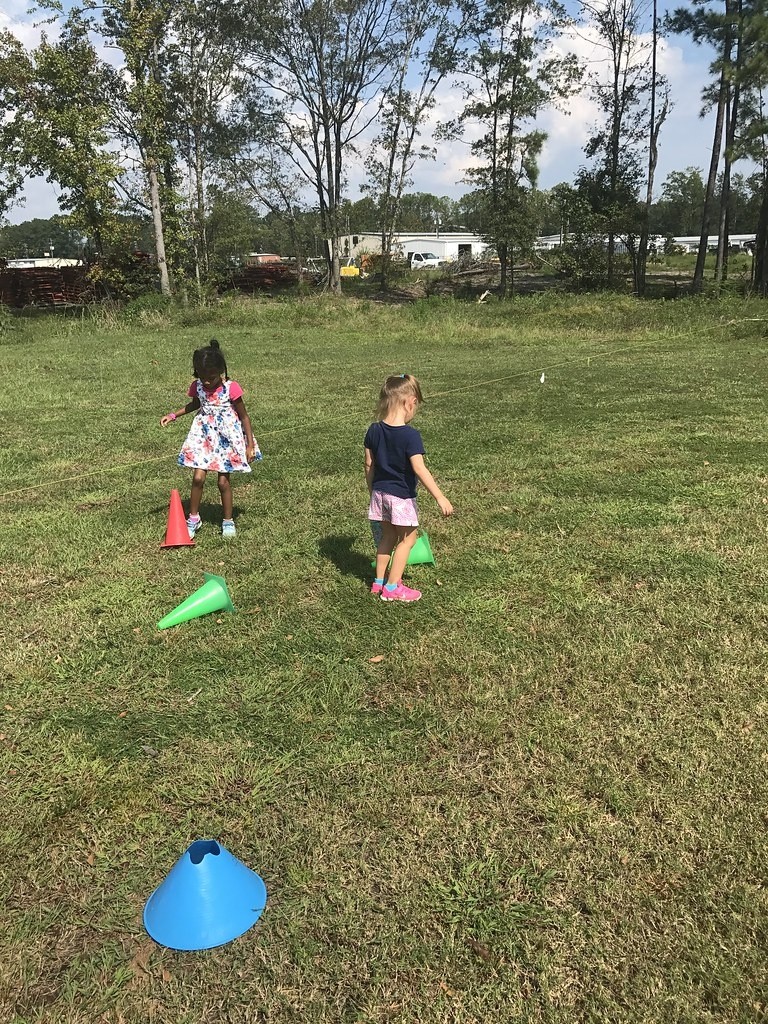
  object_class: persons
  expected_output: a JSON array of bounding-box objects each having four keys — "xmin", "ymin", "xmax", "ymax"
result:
[
  {"xmin": 363, "ymin": 375, "xmax": 454, "ymax": 601},
  {"xmin": 161, "ymin": 338, "xmax": 262, "ymax": 541}
]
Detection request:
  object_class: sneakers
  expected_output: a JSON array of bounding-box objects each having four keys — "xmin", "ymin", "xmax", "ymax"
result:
[
  {"xmin": 381, "ymin": 578, "xmax": 421, "ymax": 603},
  {"xmin": 371, "ymin": 582, "xmax": 384, "ymax": 593},
  {"xmin": 222, "ymin": 521, "xmax": 236, "ymax": 538},
  {"xmin": 186, "ymin": 516, "xmax": 202, "ymax": 540}
]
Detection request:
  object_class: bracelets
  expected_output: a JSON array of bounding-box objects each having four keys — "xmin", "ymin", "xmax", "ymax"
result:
[{"xmin": 167, "ymin": 413, "xmax": 176, "ymax": 421}]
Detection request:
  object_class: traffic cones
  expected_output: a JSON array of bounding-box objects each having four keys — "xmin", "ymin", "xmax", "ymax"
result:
[
  {"xmin": 370, "ymin": 529, "xmax": 436, "ymax": 570},
  {"xmin": 156, "ymin": 571, "xmax": 233, "ymax": 630},
  {"xmin": 160, "ymin": 489, "xmax": 196, "ymax": 547}
]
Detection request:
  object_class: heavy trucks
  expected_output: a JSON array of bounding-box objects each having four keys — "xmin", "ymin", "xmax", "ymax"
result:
[{"xmin": 393, "ymin": 251, "xmax": 448, "ymax": 278}]
